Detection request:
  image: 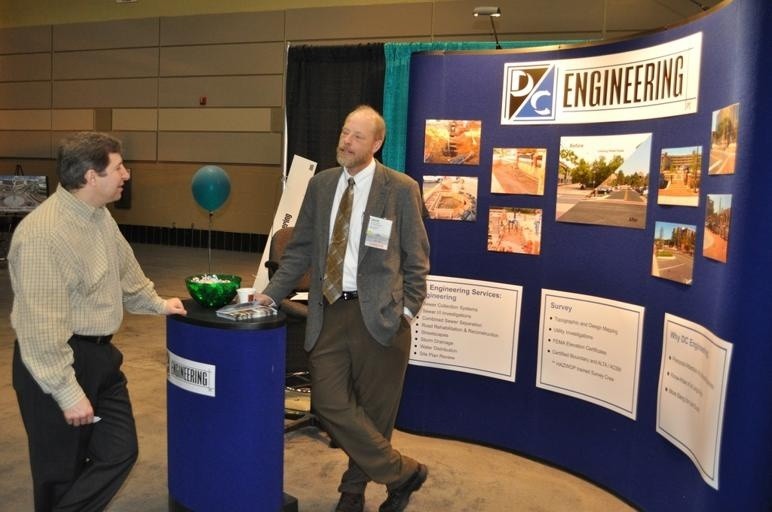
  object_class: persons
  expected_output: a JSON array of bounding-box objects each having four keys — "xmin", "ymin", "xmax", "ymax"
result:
[
  {"xmin": 253, "ymin": 105, "xmax": 431, "ymax": 510},
  {"xmin": 7, "ymin": 130, "xmax": 187, "ymax": 510}
]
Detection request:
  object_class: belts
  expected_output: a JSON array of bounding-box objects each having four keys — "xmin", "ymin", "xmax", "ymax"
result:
[
  {"xmin": 323, "ymin": 291, "xmax": 359, "ymax": 302},
  {"xmin": 71, "ymin": 335, "xmax": 113, "ymax": 345}
]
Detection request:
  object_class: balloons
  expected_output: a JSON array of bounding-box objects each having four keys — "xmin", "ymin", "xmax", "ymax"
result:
[{"xmin": 191, "ymin": 166, "xmax": 232, "ymax": 212}]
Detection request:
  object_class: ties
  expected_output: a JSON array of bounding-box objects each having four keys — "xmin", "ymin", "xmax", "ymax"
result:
[{"xmin": 321, "ymin": 177, "xmax": 355, "ymax": 305}]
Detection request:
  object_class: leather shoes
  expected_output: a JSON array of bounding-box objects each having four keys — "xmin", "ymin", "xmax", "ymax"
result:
[
  {"xmin": 378, "ymin": 463, "xmax": 428, "ymax": 512},
  {"xmin": 334, "ymin": 492, "xmax": 365, "ymax": 512}
]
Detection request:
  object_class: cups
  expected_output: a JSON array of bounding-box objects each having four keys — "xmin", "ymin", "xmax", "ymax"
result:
[{"xmin": 236, "ymin": 288, "xmax": 256, "ymax": 303}]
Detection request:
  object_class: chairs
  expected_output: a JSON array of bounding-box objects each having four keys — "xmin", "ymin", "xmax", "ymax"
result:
[{"xmin": 263, "ymin": 227, "xmax": 340, "ymax": 449}]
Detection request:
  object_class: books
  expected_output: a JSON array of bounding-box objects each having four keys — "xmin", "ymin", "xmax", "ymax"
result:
[{"xmin": 216, "ymin": 299, "xmax": 277, "ymax": 322}]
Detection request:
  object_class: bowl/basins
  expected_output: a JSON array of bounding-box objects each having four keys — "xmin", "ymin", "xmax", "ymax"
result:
[{"xmin": 184, "ymin": 275, "xmax": 242, "ymax": 307}]
